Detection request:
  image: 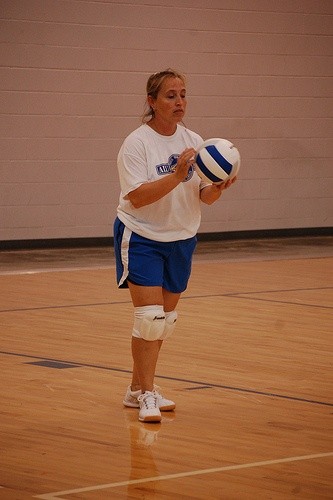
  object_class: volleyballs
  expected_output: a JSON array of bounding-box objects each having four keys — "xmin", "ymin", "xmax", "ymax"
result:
[{"xmin": 191, "ymin": 137, "xmax": 242, "ymax": 186}]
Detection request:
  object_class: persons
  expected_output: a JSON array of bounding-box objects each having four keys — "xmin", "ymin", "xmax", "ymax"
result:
[{"xmin": 112, "ymin": 67, "xmax": 239, "ymax": 423}]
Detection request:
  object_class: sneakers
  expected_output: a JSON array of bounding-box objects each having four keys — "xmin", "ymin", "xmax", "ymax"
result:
[
  {"xmin": 137, "ymin": 391, "xmax": 164, "ymax": 425},
  {"xmin": 123, "ymin": 384, "xmax": 176, "ymax": 413}
]
